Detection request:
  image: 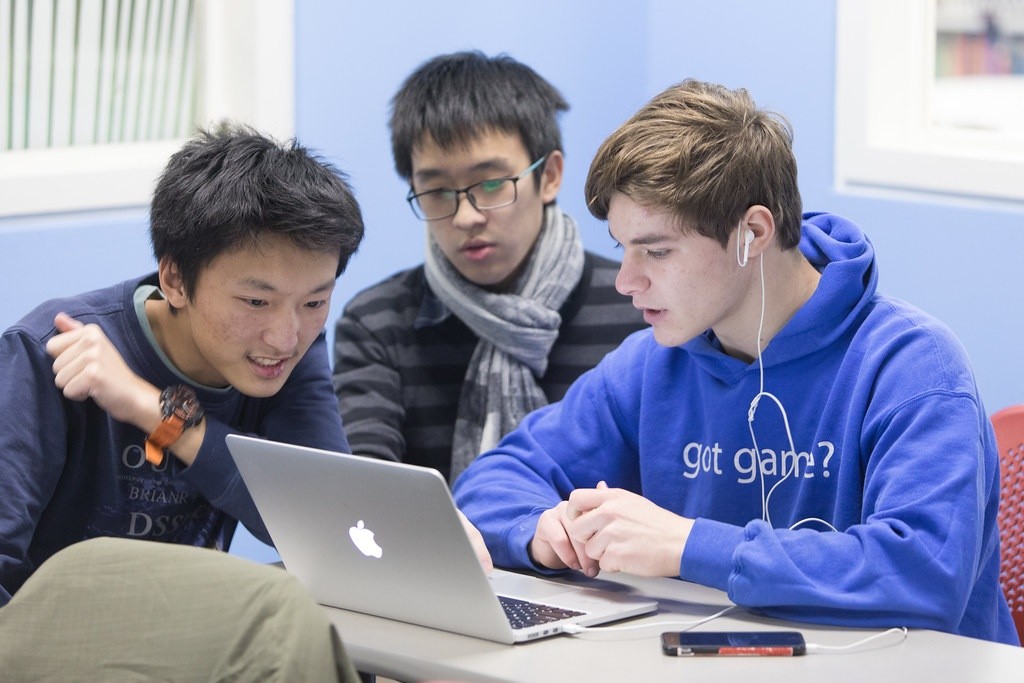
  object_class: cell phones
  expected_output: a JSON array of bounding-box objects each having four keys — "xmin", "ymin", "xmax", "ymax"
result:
[{"xmin": 660, "ymin": 631, "xmax": 804, "ymax": 656}]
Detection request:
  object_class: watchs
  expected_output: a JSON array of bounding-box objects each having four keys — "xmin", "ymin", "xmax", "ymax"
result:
[{"xmin": 145, "ymin": 384, "xmax": 203, "ymax": 465}]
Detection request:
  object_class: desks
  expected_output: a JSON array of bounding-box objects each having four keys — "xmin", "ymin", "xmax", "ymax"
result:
[{"xmin": 319, "ymin": 567, "xmax": 1024, "ymax": 683}]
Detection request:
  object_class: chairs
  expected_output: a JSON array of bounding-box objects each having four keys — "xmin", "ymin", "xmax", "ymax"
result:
[{"xmin": 988, "ymin": 405, "xmax": 1024, "ymax": 651}]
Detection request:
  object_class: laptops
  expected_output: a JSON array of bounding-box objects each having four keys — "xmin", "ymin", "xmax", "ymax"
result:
[{"xmin": 224, "ymin": 434, "xmax": 659, "ymax": 641}]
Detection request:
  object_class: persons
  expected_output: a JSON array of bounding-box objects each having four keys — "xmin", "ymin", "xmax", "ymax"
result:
[
  {"xmin": 332, "ymin": 53, "xmax": 649, "ymax": 566},
  {"xmin": 0, "ymin": 132, "xmax": 351, "ymax": 606},
  {"xmin": 452, "ymin": 80, "xmax": 1022, "ymax": 647}
]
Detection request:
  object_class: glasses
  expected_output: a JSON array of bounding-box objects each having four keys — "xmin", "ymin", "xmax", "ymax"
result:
[{"xmin": 407, "ymin": 153, "xmax": 548, "ymax": 221}]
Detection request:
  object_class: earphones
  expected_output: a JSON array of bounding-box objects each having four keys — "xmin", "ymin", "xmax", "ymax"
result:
[{"xmin": 742, "ymin": 229, "xmax": 755, "ymax": 262}]
